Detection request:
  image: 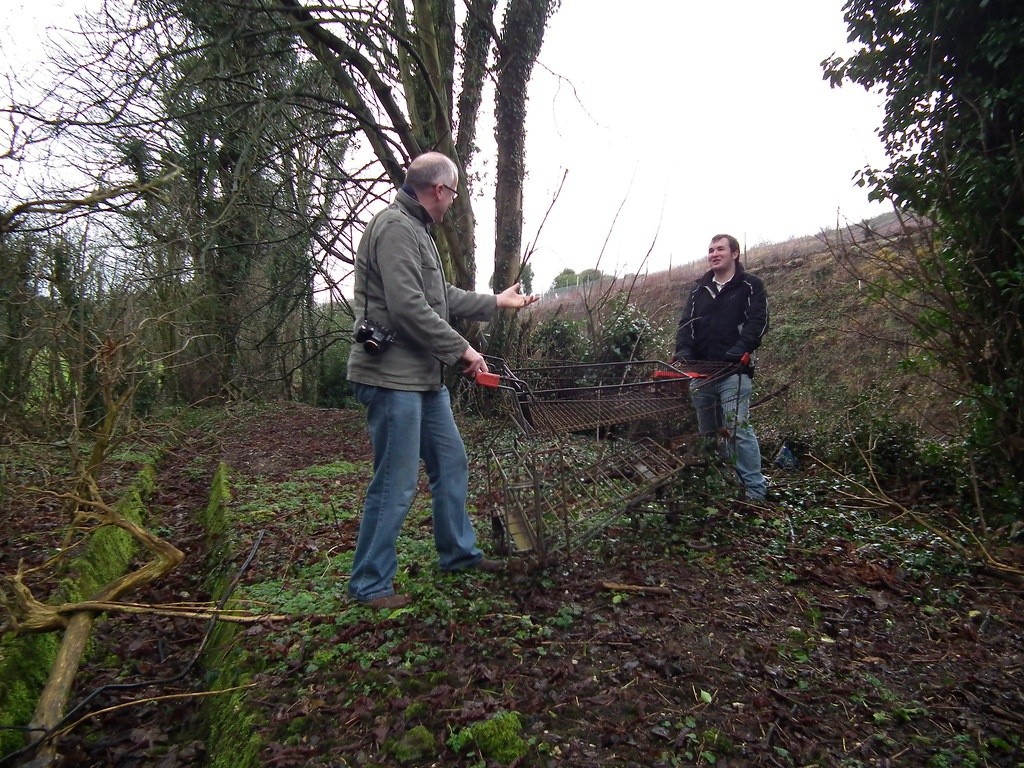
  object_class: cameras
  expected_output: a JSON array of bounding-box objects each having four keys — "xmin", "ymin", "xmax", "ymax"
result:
[{"xmin": 355, "ymin": 320, "xmax": 394, "ymax": 355}]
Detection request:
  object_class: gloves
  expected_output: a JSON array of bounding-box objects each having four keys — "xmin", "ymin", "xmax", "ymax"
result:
[
  {"xmin": 724, "ymin": 343, "xmax": 745, "ymax": 363},
  {"xmin": 674, "ymin": 346, "xmax": 693, "ymax": 364}
]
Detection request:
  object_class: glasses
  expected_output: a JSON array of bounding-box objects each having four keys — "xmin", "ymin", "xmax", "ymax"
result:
[{"xmin": 433, "ymin": 184, "xmax": 459, "ymax": 199}]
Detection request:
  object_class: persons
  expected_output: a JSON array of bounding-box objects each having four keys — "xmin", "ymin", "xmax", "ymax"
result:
[
  {"xmin": 668, "ymin": 233, "xmax": 767, "ymax": 502},
  {"xmin": 344, "ymin": 152, "xmax": 540, "ymax": 610}
]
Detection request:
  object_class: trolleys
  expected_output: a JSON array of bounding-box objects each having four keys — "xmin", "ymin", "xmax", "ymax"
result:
[{"xmin": 465, "ymin": 352, "xmax": 752, "ymax": 600}]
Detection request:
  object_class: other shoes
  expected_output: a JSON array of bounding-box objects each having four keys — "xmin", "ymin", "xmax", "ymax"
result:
[
  {"xmin": 366, "ymin": 592, "xmax": 405, "ymax": 610},
  {"xmin": 476, "ymin": 560, "xmax": 505, "ymax": 571}
]
